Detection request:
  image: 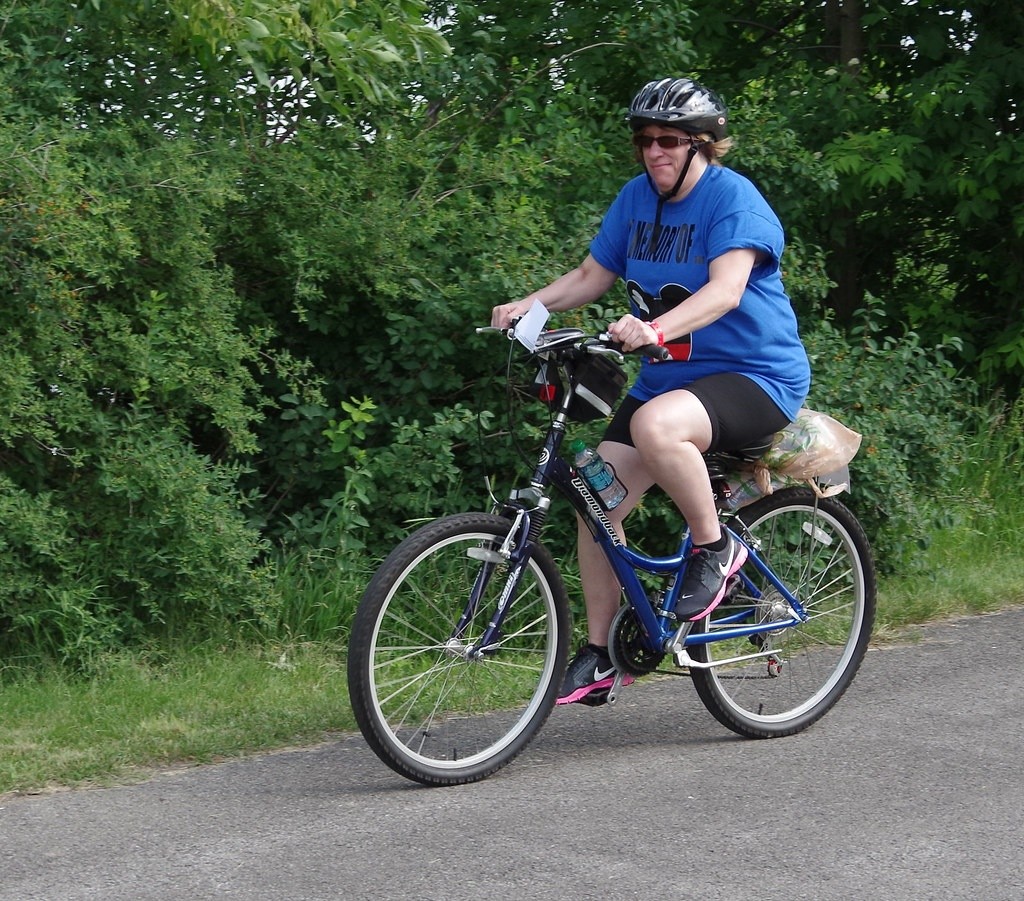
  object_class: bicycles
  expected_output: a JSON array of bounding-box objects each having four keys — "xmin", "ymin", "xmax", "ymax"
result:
[{"xmin": 346, "ymin": 318, "xmax": 878, "ymax": 790}]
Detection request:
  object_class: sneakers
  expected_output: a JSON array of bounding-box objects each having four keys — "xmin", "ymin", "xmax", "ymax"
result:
[
  {"xmin": 553, "ymin": 637, "xmax": 636, "ymax": 705},
  {"xmin": 674, "ymin": 522, "xmax": 748, "ymax": 621}
]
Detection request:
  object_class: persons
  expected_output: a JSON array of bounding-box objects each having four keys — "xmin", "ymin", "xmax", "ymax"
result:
[{"xmin": 491, "ymin": 75, "xmax": 811, "ymax": 704}]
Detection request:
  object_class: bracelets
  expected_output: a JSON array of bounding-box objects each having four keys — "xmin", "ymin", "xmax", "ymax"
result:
[{"xmin": 644, "ymin": 321, "xmax": 664, "ymax": 346}]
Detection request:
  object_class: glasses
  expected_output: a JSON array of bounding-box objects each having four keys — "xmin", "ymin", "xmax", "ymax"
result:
[{"xmin": 631, "ymin": 133, "xmax": 701, "ymax": 149}]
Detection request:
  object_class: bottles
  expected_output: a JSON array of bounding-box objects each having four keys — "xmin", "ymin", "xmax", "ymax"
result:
[{"xmin": 572, "ymin": 437, "xmax": 625, "ymax": 509}]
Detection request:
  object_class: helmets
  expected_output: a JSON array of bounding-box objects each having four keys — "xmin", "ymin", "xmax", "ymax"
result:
[{"xmin": 628, "ymin": 78, "xmax": 733, "ymax": 142}]
